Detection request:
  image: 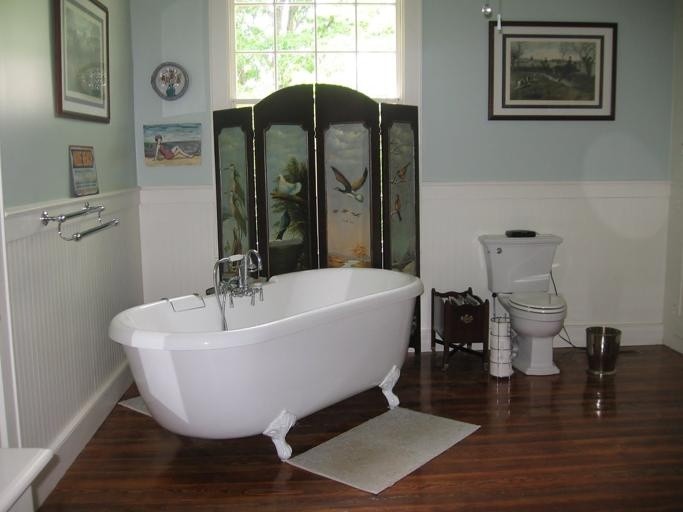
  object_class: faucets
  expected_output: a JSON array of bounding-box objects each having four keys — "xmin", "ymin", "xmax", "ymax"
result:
[{"xmin": 242, "ymin": 248, "xmax": 263, "ymax": 293}]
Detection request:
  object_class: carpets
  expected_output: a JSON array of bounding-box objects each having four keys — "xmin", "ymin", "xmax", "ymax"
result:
[
  {"xmin": 118, "ymin": 396, "xmax": 153, "ymax": 418},
  {"xmin": 285, "ymin": 406, "xmax": 482, "ymax": 495}
]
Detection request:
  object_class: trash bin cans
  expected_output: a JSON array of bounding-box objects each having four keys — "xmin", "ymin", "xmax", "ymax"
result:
[{"xmin": 585, "ymin": 326, "xmax": 621, "ymax": 375}]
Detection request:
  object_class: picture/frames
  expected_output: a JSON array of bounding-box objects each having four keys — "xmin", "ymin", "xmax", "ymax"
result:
[
  {"xmin": 54, "ymin": 0, "xmax": 110, "ymax": 124},
  {"xmin": 487, "ymin": 20, "xmax": 618, "ymax": 121}
]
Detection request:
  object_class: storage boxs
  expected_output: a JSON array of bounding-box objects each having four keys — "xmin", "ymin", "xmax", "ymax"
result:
[{"xmin": 431, "ymin": 287, "xmax": 489, "ymax": 373}]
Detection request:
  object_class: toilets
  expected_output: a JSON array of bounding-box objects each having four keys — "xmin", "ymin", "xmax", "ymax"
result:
[{"xmin": 477, "ymin": 231, "xmax": 570, "ymax": 375}]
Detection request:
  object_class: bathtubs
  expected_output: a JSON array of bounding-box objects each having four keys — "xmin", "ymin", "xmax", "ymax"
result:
[{"xmin": 108, "ymin": 267, "xmax": 426, "ymax": 461}]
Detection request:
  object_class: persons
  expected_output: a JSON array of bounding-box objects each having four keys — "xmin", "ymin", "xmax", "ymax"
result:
[{"xmin": 151, "ymin": 135, "xmax": 195, "ymax": 162}]
